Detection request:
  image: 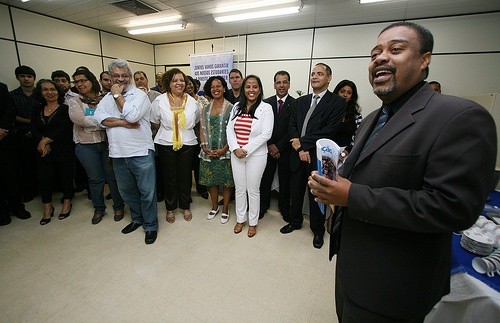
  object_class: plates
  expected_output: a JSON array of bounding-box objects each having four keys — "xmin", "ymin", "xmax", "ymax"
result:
[{"xmin": 460, "ymin": 228, "xmax": 495, "ymax": 256}]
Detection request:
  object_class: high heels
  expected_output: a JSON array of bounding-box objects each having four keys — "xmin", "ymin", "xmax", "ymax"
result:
[
  {"xmin": 248, "ymin": 226, "xmax": 256, "ymax": 237},
  {"xmin": 40, "ymin": 206, "xmax": 54, "ymax": 225},
  {"xmin": 58, "ymin": 203, "xmax": 72, "ymax": 220},
  {"xmin": 234, "ymin": 221, "xmax": 245, "ymax": 233}
]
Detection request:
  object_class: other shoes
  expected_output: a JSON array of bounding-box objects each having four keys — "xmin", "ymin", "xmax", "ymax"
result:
[
  {"xmin": 87, "ymin": 188, "xmax": 92, "ymax": 200},
  {"xmin": 182, "ymin": 209, "xmax": 192, "ymax": 221},
  {"xmin": 197, "ymin": 189, "xmax": 209, "ymax": 199},
  {"xmin": 218, "ymin": 197, "xmax": 233, "ymax": 205},
  {"xmin": 8, "ymin": 202, "xmax": 31, "ymax": 219},
  {"xmin": 221, "ymin": 209, "xmax": 229, "ymax": 223},
  {"xmin": 75, "ymin": 184, "xmax": 84, "ymax": 193},
  {"xmin": 186, "ymin": 194, "xmax": 193, "ymax": 203},
  {"xmin": 157, "ymin": 191, "xmax": 163, "ymax": 202},
  {"xmin": 207, "ymin": 206, "xmax": 219, "ymax": 220},
  {"xmin": 166, "ymin": 210, "xmax": 175, "ymax": 223},
  {"xmin": 279, "ymin": 207, "xmax": 292, "ymax": 222},
  {"xmin": 259, "ymin": 209, "xmax": 267, "ymax": 220},
  {"xmin": 114, "ymin": 209, "xmax": 124, "ymax": 221},
  {"xmin": 92, "ymin": 213, "xmax": 104, "ymax": 224},
  {"xmin": 0, "ymin": 201, "xmax": 11, "ymax": 226},
  {"xmin": 106, "ymin": 193, "xmax": 112, "ymax": 200}
]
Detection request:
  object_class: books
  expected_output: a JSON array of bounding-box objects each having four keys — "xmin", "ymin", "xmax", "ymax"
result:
[{"xmin": 317, "ymin": 138, "xmax": 347, "ymax": 215}]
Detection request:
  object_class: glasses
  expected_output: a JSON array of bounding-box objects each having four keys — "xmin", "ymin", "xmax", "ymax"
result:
[
  {"xmin": 72, "ymin": 78, "xmax": 88, "ymax": 84},
  {"xmin": 274, "ymin": 80, "xmax": 290, "ymax": 84}
]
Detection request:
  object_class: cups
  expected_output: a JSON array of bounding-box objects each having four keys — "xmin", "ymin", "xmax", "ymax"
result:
[
  {"xmin": 472, "ymin": 249, "xmax": 500, "ymax": 277},
  {"xmin": 475, "ymin": 216, "xmax": 500, "ymax": 249}
]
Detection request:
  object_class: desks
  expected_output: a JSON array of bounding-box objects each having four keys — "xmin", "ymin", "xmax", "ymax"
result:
[{"xmin": 423, "ymin": 192, "xmax": 500, "ymax": 323}]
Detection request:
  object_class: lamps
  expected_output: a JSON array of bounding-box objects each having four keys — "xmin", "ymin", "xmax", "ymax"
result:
[
  {"xmin": 214, "ymin": 0, "xmax": 304, "ymax": 24},
  {"xmin": 126, "ymin": 19, "xmax": 188, "ymax": 36}
]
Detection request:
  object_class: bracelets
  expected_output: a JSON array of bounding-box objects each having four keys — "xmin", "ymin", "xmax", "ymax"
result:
[{"xmin": 113, "ymin": 94, "xmax": 122, "ymax": 100}]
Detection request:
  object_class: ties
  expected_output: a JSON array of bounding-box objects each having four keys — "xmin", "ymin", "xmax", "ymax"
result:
[
  {"xmin": 301, "ymin": 95, "xmax": 320, "ymax": 138},
  {"xmin": 278, "ymin": 100, "xmax": 283, "ymax": 114},
  {"xmin": 333, "ymin": 105, "xmax": 391, "ymax": 234}
]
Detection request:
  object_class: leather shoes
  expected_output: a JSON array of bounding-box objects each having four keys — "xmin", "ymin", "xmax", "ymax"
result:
[
  {"xmin": 122, "ymin": 222, "xmax": 142, "ymax": 234},
  {"xmin": 145, "ymin": 230, "xmax": 157, "ymax": 244},
  {"xmin": 313, "ymin": 235, "xmax": 323, "ymax": 248},
  {"xmin": 281, "ymin": 224, "xmax": 302, "ymax": 234}
]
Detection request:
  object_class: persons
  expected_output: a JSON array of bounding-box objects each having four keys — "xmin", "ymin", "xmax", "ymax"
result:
[
  {"xmin": 429, "ymin": 81, "xmax": 441, "ymax": 93},
  {"xmin": 0, "ymin": 59, "xmax": 362, "ymax": 249},
  {"xmin": 309, "ymin": 22, "xmax": 497, "ymax": 323}
]
